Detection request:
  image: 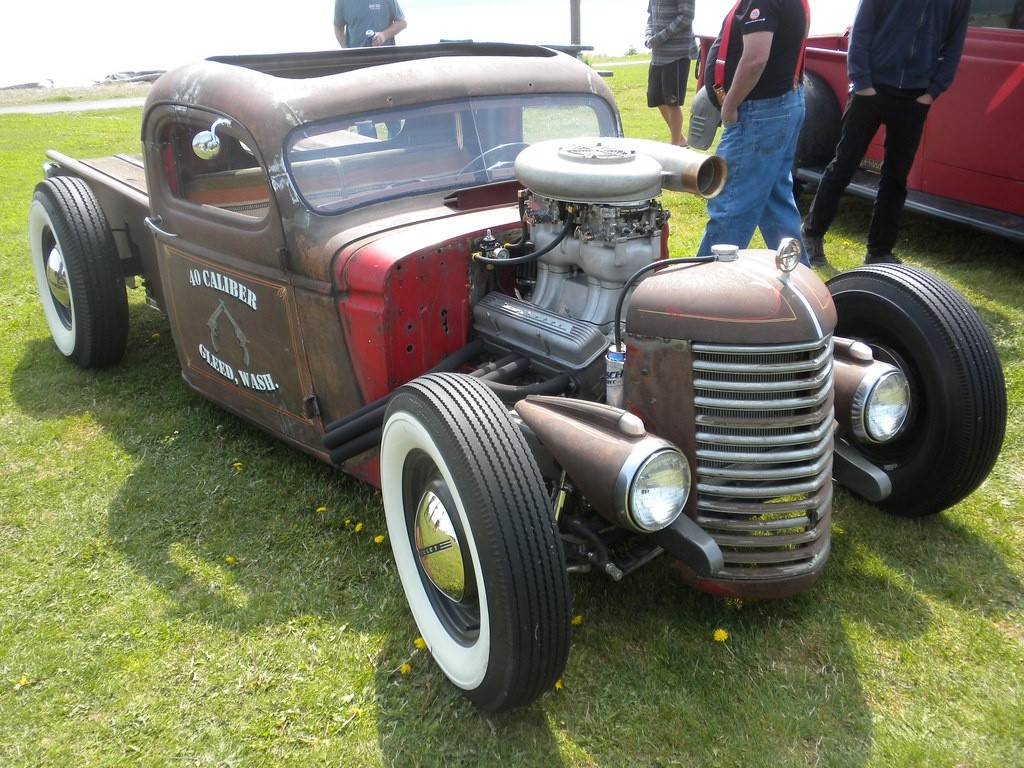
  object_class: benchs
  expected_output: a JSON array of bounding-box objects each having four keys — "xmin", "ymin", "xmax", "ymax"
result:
[{"xmin": 187, "ymin": 142, "xmax": 473, "ymax": 217}]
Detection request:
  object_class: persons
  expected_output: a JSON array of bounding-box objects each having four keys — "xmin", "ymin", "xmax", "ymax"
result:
[
  {"xmin": 333, "ymin": 0, "xmax": 408, "ymax": 49},
  {"xmin": 642, "ymin": 0, "xmax": 972, "ymax": 266}
]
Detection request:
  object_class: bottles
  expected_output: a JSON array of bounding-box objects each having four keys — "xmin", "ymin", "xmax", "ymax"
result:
[{"xmin": 366, "ymin": 29, "xmax": 378, "ymax": 45}]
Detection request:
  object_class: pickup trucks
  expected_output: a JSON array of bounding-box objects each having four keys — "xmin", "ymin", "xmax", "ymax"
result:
[
  {"xmin": 31, "ymin": 46, "xmax": 1006, "ymax": 715},
  {"xmin": 791, "ymin": 0, "xmax": 1024, "ymax": 243}
]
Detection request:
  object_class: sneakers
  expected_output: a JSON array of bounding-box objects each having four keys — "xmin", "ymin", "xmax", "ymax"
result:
[{"xmin": 797, "ymin": 221, "xmax": 901, "ymax": 265}]
